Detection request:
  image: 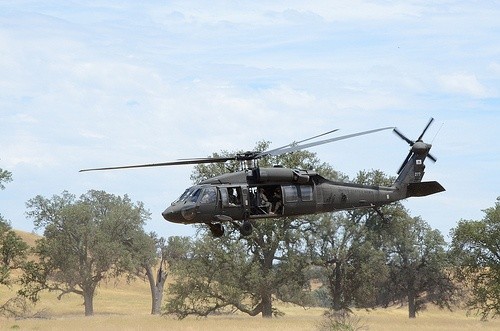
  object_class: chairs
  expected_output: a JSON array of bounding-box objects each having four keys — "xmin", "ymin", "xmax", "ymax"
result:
[{"xmin": 254, "ymin": 192, "xmax": 269, "ymax": 216}]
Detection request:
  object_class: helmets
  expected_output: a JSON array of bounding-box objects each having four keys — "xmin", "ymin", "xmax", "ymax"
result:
[{"xmin": 259, "ymin": 188, "xmax": 264, "ymax": 191}]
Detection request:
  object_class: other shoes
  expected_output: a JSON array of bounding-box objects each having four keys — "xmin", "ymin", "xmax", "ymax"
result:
[{"xmin": 268, "ymin": 212, "xmax": 275, "ymax": 215}]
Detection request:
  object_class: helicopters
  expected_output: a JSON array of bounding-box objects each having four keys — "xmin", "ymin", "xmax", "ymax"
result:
[{"xmin": 77, "ymin": 116, "xmax": 445, "ymax": 236}]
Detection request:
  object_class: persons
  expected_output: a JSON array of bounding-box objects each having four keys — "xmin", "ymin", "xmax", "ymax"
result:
[
  {"xmin": 203, "ymin": 189, "xmax": 216, "ymax": 203},
  {"xmin": 191, "ymin": 192, "xmax": 198, "ymax": 202},
  {"xmin": 273, "ymin": 187, "xmax": 283, "ymax": 214},
  {"xmin": 236, "ymin": 187, "xmax": 242, "ymax": 204},
  {"xmin": 259, "ymin": 187, "xmax": 275, "ymax": 215},
  {"xmin": 227, "ymin": 188, "xmax": 238, "ymax": 203}
]
